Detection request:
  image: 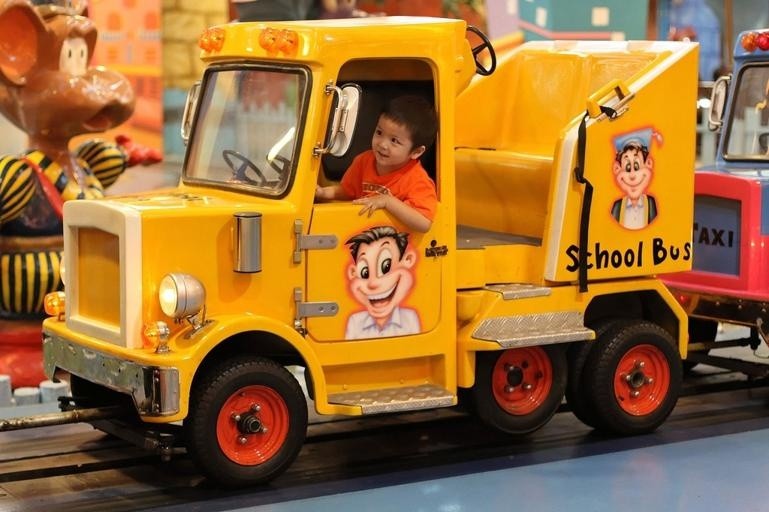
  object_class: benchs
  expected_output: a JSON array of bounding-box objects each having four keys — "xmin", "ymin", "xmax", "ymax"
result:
[{"xmin": 452, "ymin": 40, "xmax": 696, "ymax": 238}]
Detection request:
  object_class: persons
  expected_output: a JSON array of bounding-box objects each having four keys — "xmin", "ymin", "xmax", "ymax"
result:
[{"xmin": 314, "ymin": 95, "xmax": 437, "ymax": 233}]
